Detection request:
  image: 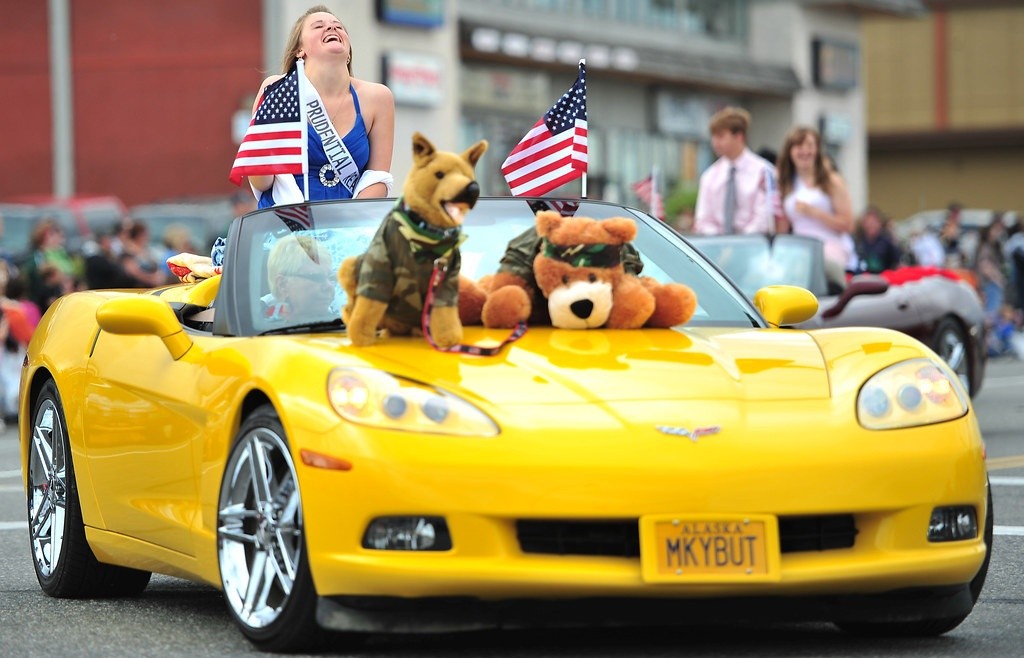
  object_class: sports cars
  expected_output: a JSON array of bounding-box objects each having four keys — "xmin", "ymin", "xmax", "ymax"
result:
[
  {"xmin": 14, "ymin": 190, "xmax": 997, "ymax": 656},
  {"xmin": 671, "ymin": 230, "xmax": 989, "ymax": 402}
]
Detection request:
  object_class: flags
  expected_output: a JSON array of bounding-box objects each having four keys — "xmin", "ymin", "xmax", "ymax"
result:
[
  {"xmin": 634, "ymin": 176, "xmax": 665, "ymax": 222},
  {"xmin": 526, "ymin": 199, "xmax": 580, "ymax": 218},
  {"xmin": 273, "ymin": 205, "xmax": 318, "ymax": 263},
  {"xmin": 228, "ymin": 71, "xmax": 303, "ymax": 186},
  {"xmin": 501, "ymin": 62, "xmax": 588, "ymax": 198}
]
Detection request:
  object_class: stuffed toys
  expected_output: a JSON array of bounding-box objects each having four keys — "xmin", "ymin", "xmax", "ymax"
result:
[
  {"xmin": 479, "ymin": 210, "xmax": 696, "ymax": 330},
  {"xmin": 338, "ymin": 131, "xmax": 488, "ymax": 345}
]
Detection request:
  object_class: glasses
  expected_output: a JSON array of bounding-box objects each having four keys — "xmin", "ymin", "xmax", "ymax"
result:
[{"xmin": 284, "ymin": 271, "xmax": 338, "ymax": 283}]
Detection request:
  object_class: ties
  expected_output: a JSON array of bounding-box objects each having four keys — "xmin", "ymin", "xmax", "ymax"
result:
[{"xmin": 725, "ymin": 166, "xmax": 736, "ymax": 236}]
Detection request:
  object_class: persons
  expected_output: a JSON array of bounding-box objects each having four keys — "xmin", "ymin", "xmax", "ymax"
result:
[
  {"xmin": 695, "ymin": 106, "xmax": 791, "ymax": 235},
  {"xmin": 0, "ymin": 216, "xmax": 238, "ymax": 424},
  {"xmin": 851, "ymin": 201, "xmax": 1024, "ymax": 358},
  {"xmin": 247, "ymin": 6, "xmax": 395, "ymax": 210},
  {"xmin": 190, "ymin": 236, "xmax": 341, "ymax": 331},
  {"xmin": 758, "ymin": 126, "xmax": 858, "ymax": 280}
]
